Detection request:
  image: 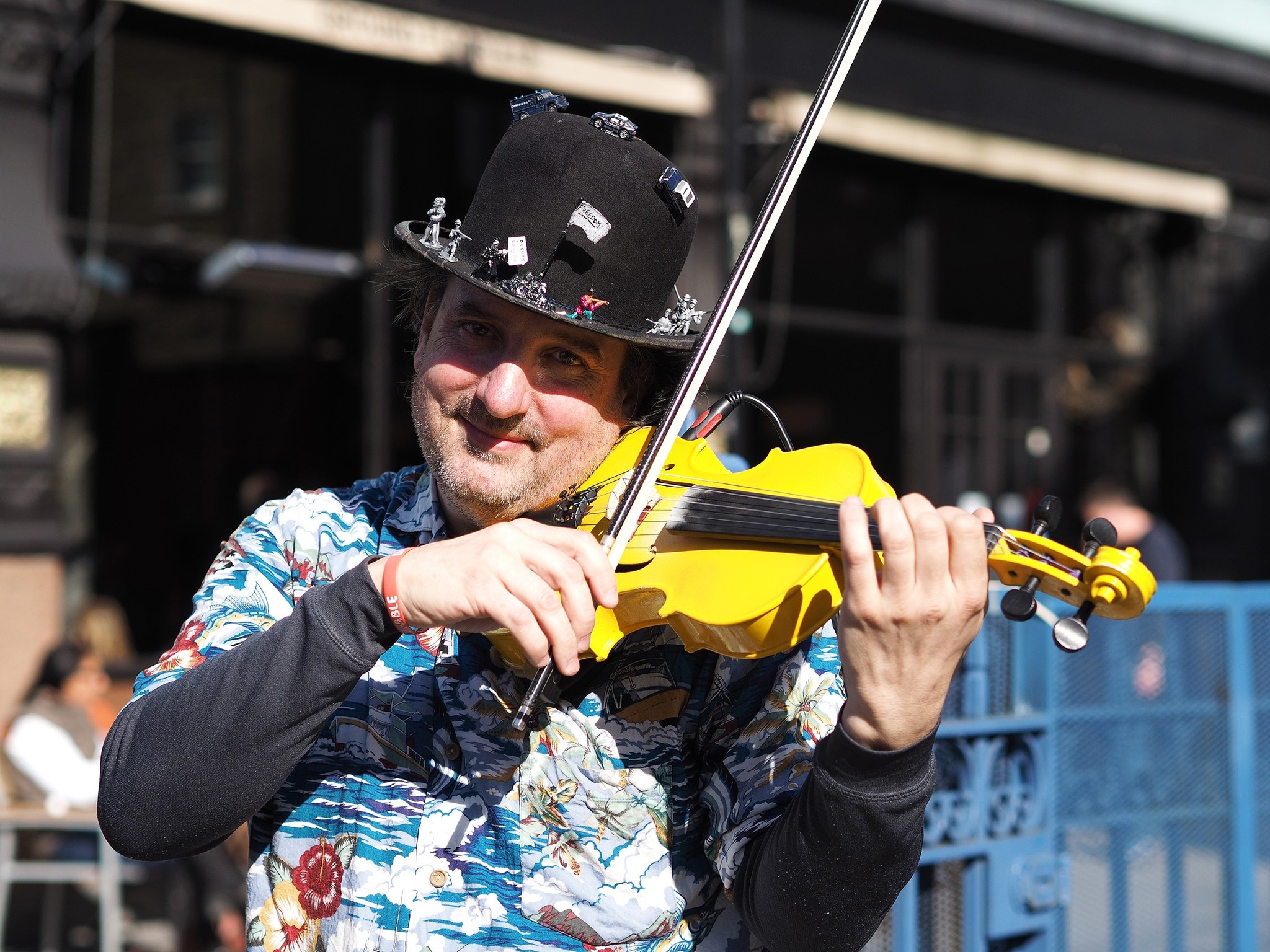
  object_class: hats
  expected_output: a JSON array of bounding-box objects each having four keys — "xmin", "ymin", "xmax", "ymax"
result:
[{"xmin": 386, "ymin": 108, "xmax": 707, "ymax": 351}]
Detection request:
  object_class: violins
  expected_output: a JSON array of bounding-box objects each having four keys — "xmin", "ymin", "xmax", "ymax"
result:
[{"xmin": 484, "ymin": 418, "xmax": 1160, "ymax": 670}]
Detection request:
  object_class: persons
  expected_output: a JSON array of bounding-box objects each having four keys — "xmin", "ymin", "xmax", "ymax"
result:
[
  {"xmin": 671, "ymin": 294, "xmax": 703, "ymax": 335},
  {"xmin": 555, "ymin": 287, "xmax": 610, "ymax": 323},
  {"xmin": 420, "ymin": 196, "xmax": 445, "ymax": 251},
  {"xmin": 95, "ymin": 92, "xmax": 990, "ymax": 952},
  {"xmin": 646, "ymin": 308, "xmax": 671, "ymax": 334},
  {"xmin": 0, "ymin": 591, "xmax": 251, "ymax": 952},
  {"xmin": 1078, "ymin": 477, "xmax": 1192, "ymax": 603},
  {"xmin": 441, "ymin": 219, "xmax": 472, "ymax": 262},
  {"xmin": 470, "ymin": 238, "xmax": 506, "ymax": 284}
]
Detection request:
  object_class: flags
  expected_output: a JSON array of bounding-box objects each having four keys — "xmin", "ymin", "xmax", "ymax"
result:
[
  {"xmin": 506, "ymin": 236, "xmax": 529, "ymax": 267},
  {"xmin": 566, "ymin": 199, "xmax": 612, "ymax": 245}
]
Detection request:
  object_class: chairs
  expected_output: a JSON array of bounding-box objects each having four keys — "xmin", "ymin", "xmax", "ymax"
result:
[{"xmin": 0, "ymin": 738, "xmax": 149, "ymax": 952}]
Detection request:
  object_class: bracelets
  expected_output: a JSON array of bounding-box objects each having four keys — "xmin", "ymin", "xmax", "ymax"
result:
[{"xmin": 384, "ymin": 545, "xmax": 417, "ymax": 643}]
